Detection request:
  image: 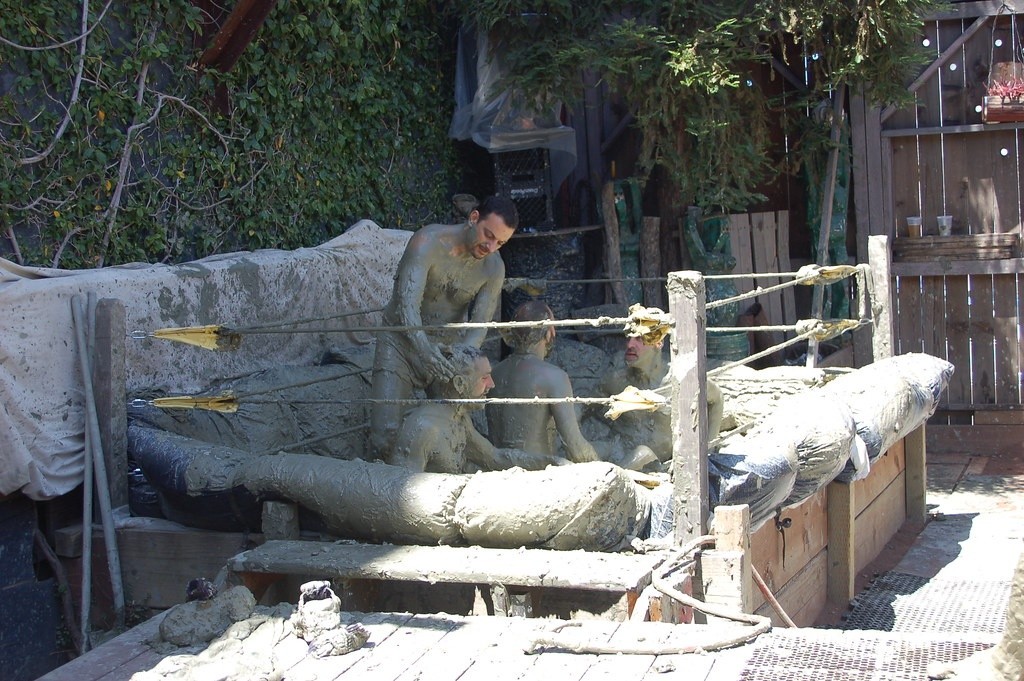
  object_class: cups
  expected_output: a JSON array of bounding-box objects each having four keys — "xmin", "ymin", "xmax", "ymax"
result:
[
  {"xmin": 906, "ymin": 217, "xmax": 922, "ymax": 237},
  {"xmin": 936, "ymin": 216, "xmax": 953, "ymax": 236}
]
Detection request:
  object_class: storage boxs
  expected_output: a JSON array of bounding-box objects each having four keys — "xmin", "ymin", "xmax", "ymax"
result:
[{"xmin": 477, "ymin": 148, "xmax": 558, "ymax": 233}]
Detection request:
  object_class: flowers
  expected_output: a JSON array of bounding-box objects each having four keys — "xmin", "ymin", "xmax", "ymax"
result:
[{"xmin": 983, "ymin": 74, "xmax": 1024, "ymax": 105}]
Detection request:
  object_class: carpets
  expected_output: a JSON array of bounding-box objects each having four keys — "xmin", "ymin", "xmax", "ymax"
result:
[
  {"xmin": 736, "ymin": 633, "xmax": 996, "ymax": 681},
  {"xmin": 831, "ymin": 572, "xmax": 1013, "ymax": 634}
]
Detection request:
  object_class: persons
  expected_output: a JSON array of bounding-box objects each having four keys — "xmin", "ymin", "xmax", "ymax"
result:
[
  {"xmin": 486, "ymin": 302, "xmax": 598, "ymax": 463},
  {"xmin": 391, "ymin": 345, "xmax": 509, "ymax": 474},
  {"xmin": 370, "ymin": 199, "xmax": 520, "ymax": 465},
  {"xmin": 589, "ymin": 333, "xmax": 724, "ymax": 470}
]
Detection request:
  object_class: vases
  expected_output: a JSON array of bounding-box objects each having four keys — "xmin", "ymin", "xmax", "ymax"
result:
[{"xmin": 981, "ymin": 96, "xmax": 1024, "ymax": 122}]
